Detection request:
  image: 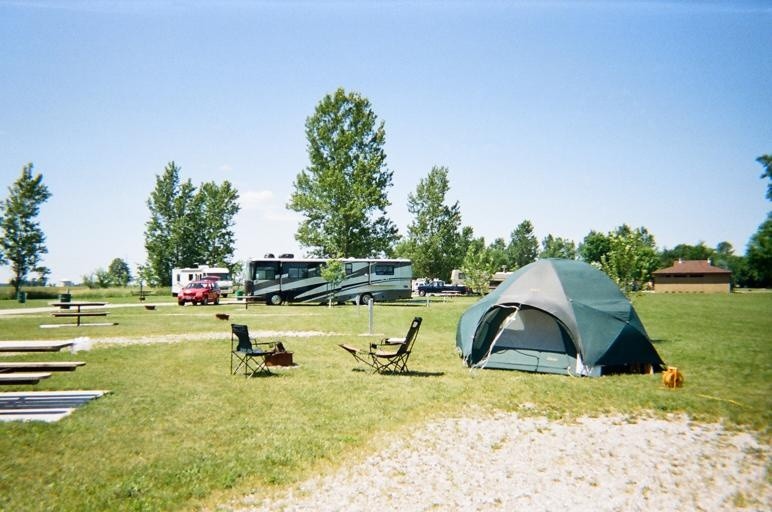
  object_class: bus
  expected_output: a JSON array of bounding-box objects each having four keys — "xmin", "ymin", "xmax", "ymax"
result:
[{"xmin": 244, "ymin": 253, "xmax": 412, "ymax": 305}]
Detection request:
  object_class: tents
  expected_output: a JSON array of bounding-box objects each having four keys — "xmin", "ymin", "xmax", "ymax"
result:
[{"xmin": 454, "ymin": 256, "xmax": 666, "ymax": 378}]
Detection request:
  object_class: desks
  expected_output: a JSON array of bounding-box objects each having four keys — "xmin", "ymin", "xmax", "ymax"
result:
[
  {"xmin": 52, "ymin": 302, "xmax": 105, "ymax": 325},
  {"xmin": 0, "ymin": 340, "xmax": 74, "ymax": 352}
]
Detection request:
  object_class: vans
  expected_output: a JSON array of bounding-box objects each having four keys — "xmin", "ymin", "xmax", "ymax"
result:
[{"xmin": 414, "ymin": 282, "xmax": 426, "ymax": 292}]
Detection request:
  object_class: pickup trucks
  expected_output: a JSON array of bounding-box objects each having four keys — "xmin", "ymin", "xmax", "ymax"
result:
[{"xmin": 418, "ymin": 280, "xmax": 467, "ymax": 296}]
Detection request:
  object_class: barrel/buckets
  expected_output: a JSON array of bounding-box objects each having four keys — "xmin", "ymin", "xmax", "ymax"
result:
[
  {"xmin": 59, "ymin": 294, "xmax": 71, "ymax": 308},
  {"xmin": 19, "ymin": 292, "xmax": 25, "ymax": 302}
]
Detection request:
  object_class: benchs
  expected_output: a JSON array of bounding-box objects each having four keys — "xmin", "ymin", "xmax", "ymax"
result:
[
  {"xmin": 0, "ymin": 359, "xmax": 86, "ymax": 373},
  {"xmin": 53, "ymin": 313, "xmax": 106, "ymax": 326},
  {"xmin": 0, "ymin": 373, "xmax": 52, "ymax": 385}
]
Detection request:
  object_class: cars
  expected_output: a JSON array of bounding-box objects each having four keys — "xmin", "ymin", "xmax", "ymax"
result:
[{"xmin": 179, "ymin": 280, "xmax": 220, "ymax": 305}]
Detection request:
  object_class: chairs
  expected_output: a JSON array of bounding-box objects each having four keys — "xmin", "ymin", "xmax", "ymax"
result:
[
  {"xmin": 338, "ymin": 316, "xmax": 423, "ymax": 374},
  {"xmin": 231, "ymin": 324, "xmax": 276, "ymax": 379}
]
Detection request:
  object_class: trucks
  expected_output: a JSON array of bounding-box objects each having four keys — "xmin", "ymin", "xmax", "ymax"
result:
[{"xmin": 172, "ymin": 264, "xmax": 233, "ymax": 297}]
